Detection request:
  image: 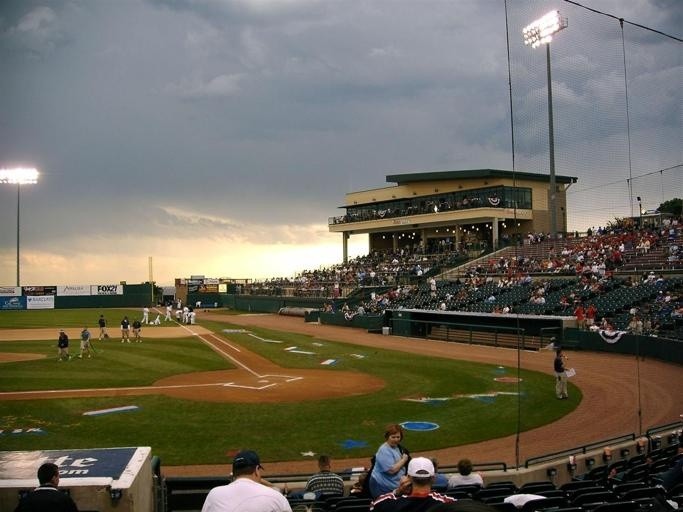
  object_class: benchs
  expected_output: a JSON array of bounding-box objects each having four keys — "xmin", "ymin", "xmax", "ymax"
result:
[{"xmin": 479, "ymin": 228, "xmax": 682, "ymax": 272}]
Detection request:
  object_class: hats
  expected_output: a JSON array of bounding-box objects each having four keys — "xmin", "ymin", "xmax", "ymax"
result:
[
  {"xmin": 233, "ymin": 450, "xmax": 264, "ymax": 471},
  {"xmin": 408, "ymin": 458, "xmax": 435, "ymax": 477}
]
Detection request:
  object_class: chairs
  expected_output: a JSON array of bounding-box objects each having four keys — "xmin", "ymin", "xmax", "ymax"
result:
[
  {"xmin": 158, "ymin": 438, "xmax": 682, "ymax": 512},
  {"xmin": 345, "ymin": 274, "xmax": 682, "ymax": 341}
]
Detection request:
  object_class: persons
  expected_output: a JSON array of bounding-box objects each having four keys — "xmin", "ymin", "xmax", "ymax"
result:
[
  {"xmin": 58, "ymin": 298, "xmax": 196, "ymax": 363},
  {"xmin": 238, "ymin": 194, "xmax": 682, "ymax": 334},
  {"xmin": 12, "ymin": 463, "xmax": 78, "ymax": 511},
  {"xmin": 201, "ymin": 450, "xmax": 294, "ymax": 512},
  {"xmin": 257, "ymin": 479, "xmax": 274, "ymax": 488},
  {"xmin": 305, "ymin": 455, "xmax": 344, "ymax": 494},
  {"xmin": 351, "ymin": 424, "xmax": 484, "ymax": 512},
  {"xmin": 554, "ymin": 350, "xmax": 568, "ymax": 400}
]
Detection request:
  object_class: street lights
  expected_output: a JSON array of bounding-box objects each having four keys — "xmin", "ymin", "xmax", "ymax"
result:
[
  {"xmin": 636, "ymin": 196, "xmax": 642, "ymax": 230},
  {"xmin": 559, "ymin": 207, "xmax": 566, "ymax": 240},
  {"xmin": 0, "ymin": 167, "xmax": 38, "ymax": 287},
  {"xmin": 521, "ymin": 8, "xmax": 568, "ymax": 240}
]
